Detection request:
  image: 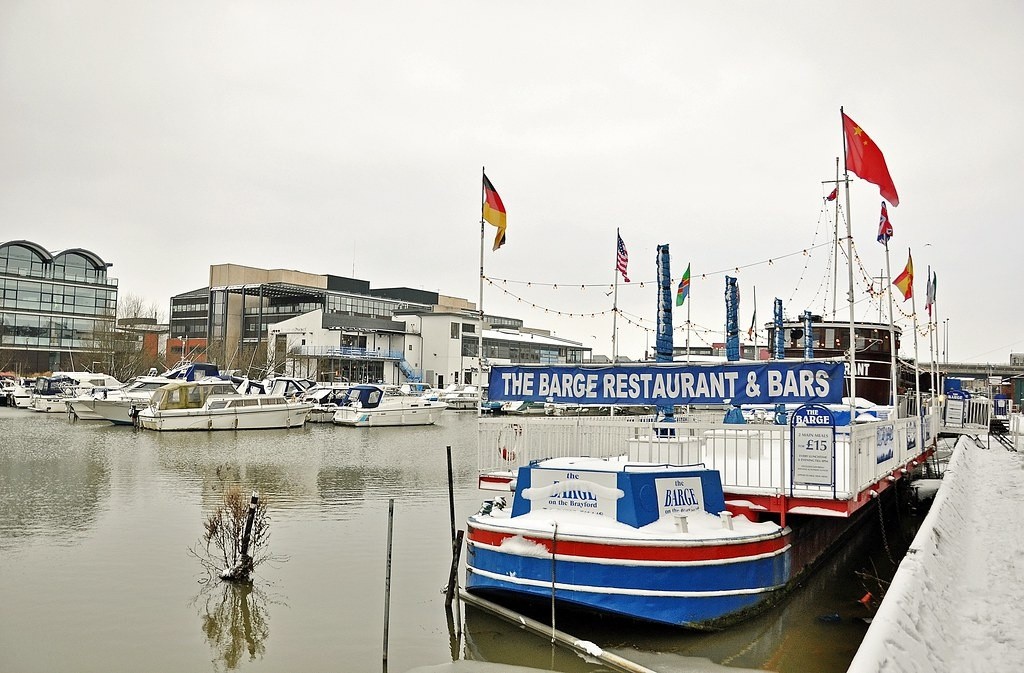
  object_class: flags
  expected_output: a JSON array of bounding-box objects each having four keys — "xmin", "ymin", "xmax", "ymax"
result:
[
  {"xmin": 841, "ymin": 113, "xmax": 899, "ymax": 208},
  {"xmin": 892, "ymin": 254, "xmax": 913, "ymax": 302},
  {"xmin": 925, "ymin": 272, "xmax": 937, "ymax": 317},
  {"xmin": 483, "ymin": 174, "xmax": 507, "ymax": 251},
  {"xmin": 616, "ymin": 235, "xmax": 630, "ymax": 282},
  {"xmin": 827, "ymin": 188, "xmax": 837, "ymax": 201},
  {"xmin": 877, "ymin": 202, "xmax": 893, "ymax": 246},
  {"xmin": 676, "ymin": 265, "xmax": 688, "ymax": 307},
  {"xmin": 747, "ymin": 311, "xmax": 756, "ymax": 341}
]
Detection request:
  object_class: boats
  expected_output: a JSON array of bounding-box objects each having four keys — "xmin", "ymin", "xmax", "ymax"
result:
[
  {"xmin": 462, "ymin": 457, "xmax": 791, "ymax": 631},
  {"xmin": 0, "ymin": 336, "xmax": 477, "ymax": 433},
  {"xmin": 477, "ymin": 157, "xmax": 939, "ymax": 522}
]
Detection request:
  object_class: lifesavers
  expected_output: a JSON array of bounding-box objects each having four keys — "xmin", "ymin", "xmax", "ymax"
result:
[{"xmin": 497, "ymin": 424, "xmax": 523, "ymax": 462}]
